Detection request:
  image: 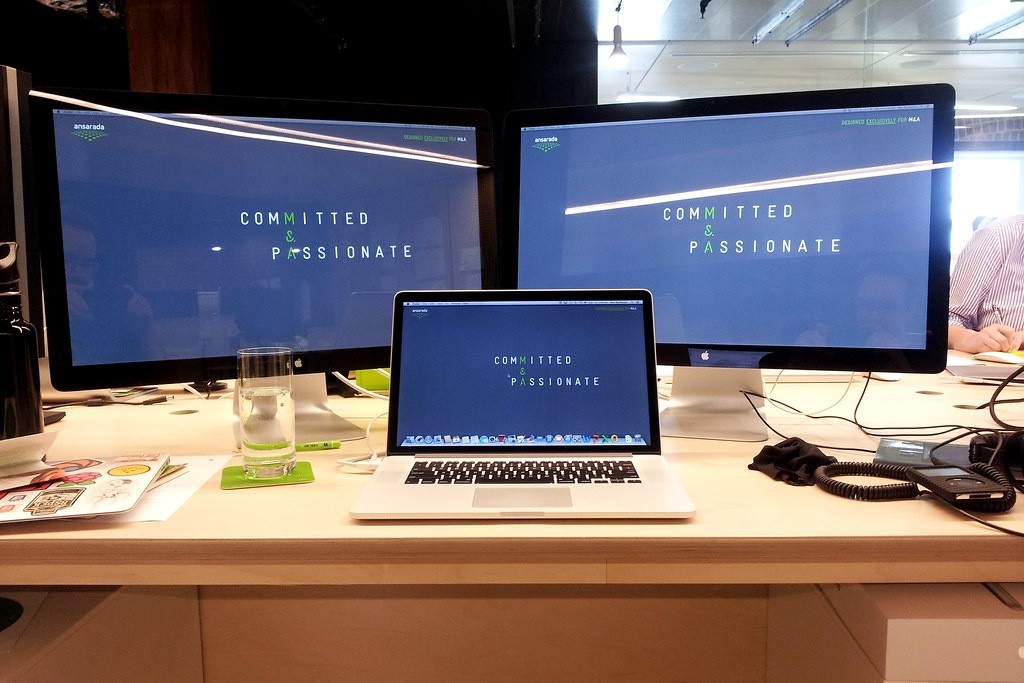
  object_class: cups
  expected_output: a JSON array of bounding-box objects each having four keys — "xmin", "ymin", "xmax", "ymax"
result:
[{"xmin": 236, "ymin": 346, "xmax": 296, "ymax": 480}]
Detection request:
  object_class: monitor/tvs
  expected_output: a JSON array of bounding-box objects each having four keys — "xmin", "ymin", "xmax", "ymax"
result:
[
  {"xmin": 27, "ymin": 89, "xmax": 492, "ymax": 449},
  {"xmin": 499, "ymin": 83, "xmax": 956, "ymax": 441}
]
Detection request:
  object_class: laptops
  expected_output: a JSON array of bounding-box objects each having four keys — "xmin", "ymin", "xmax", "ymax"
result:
[{"xmin": 349, "ymin": 290, "xmax": 696, "ymax": 520}]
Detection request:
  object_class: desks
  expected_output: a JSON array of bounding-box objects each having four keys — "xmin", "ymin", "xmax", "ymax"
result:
[{"xmin": 0, "ymin": 351, "xmax": 1024, "ymax": 683}]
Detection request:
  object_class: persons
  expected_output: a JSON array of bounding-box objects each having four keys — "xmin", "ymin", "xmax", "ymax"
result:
[{"xmin": 948, "ymin": 215, "xmax": 1024, "ymax": 353}]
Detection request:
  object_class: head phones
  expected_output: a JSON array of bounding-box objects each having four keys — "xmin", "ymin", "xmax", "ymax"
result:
[{"xmin": 969, "ymin": 430, "xmax": 1024, "ymax": 487}]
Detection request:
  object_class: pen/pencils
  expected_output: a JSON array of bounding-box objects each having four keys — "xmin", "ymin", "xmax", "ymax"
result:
[
  {"xmin": 294, "ymin": 439, "xmax": 343, "ymax": 453},
  {"xmin": 991, "ymin": 304, "xmax": 1011, "ymax": 352}
]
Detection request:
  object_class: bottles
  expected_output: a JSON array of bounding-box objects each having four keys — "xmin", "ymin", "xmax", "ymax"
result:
[{"xmin": 0, "ymin": 292, "xmax": 45, "ymax": 442}]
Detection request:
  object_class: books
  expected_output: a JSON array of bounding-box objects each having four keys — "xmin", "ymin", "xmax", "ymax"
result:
[{"xmin": 0, "ymin": 454, "xmax": 188, "ymax": 523}]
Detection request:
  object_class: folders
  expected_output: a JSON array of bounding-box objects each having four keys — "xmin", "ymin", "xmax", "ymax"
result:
[{"xmin": 945, "ymin": 364, "xmax": 1024, "ymax": 386}]
